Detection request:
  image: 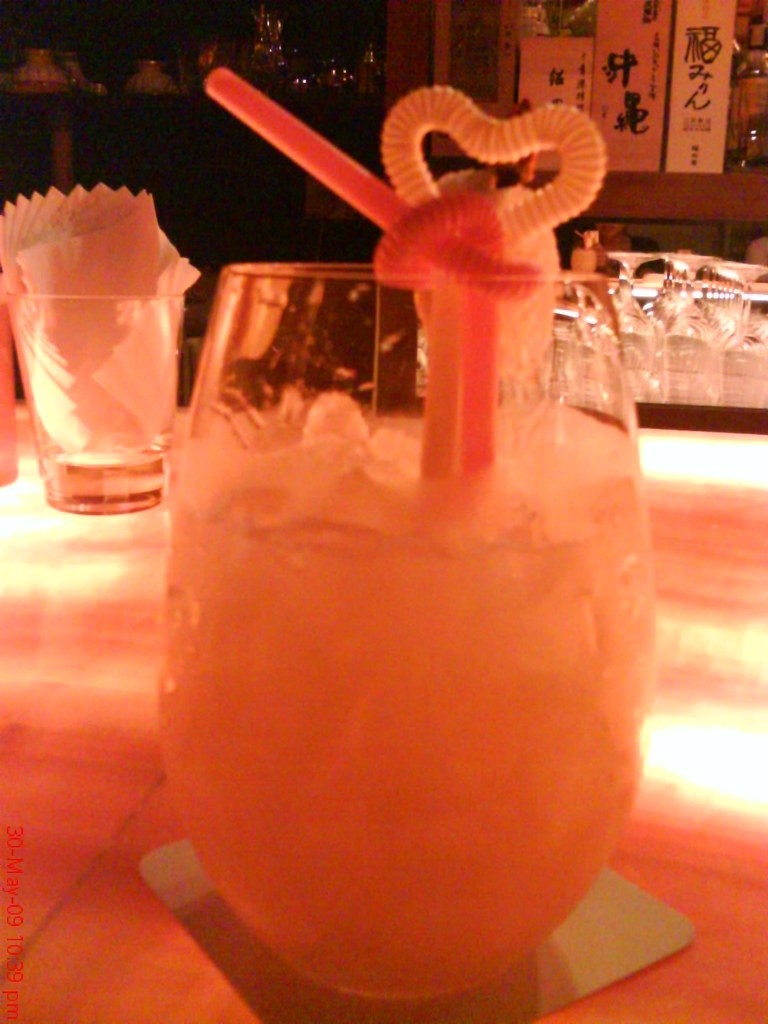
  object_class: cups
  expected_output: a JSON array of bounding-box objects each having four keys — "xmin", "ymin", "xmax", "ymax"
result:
[
  {"xmin": 5, "ymin": 290, "xmax": 184, "ymax": 515},
  {"xmin": 159, "ymin": 261, "xmax": 656, "ymax": 998}
]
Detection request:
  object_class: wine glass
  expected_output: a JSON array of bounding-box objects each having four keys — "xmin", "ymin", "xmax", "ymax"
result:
[{"xmin": 606, "ymin": 252, "xmax": 768, "ymax": 409}]
